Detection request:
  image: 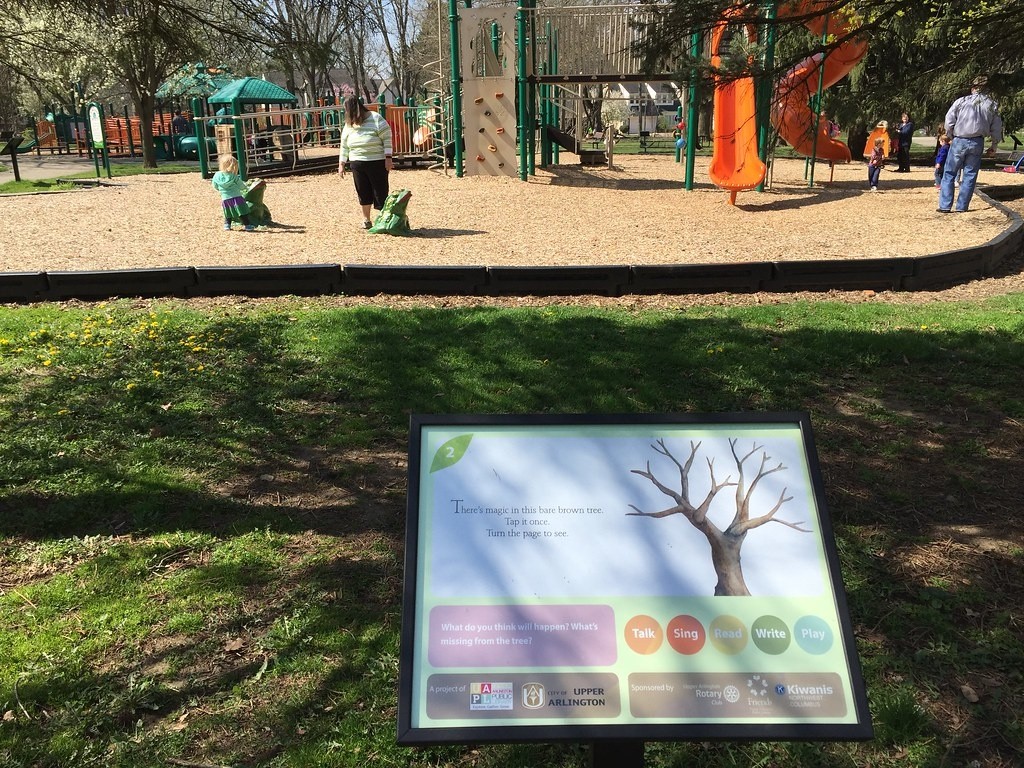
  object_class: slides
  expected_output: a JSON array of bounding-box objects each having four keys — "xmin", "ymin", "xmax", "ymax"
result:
[
  {"xmin": 16, "ymin": 133, "xmax": 53, "ymax": 152},
  {"xmin": 710, "ymin": 54, "xmax": 767, "ymax": 192},
  {"xmin": 770, "ymin": 0, "xmax": 870, "ymax": 166}
]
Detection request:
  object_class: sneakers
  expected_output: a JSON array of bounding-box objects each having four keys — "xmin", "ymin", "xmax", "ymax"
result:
[
  {"xmin": 244, "ymin": 225, "xmax": 254, "ymax": 231},
  {"xmin": 225, "ymin": 224, "xmax": 231, "ymax": 231},
  {"xmin": 362, "ymin": 220, "xmax": 372, "ymax": 228}
]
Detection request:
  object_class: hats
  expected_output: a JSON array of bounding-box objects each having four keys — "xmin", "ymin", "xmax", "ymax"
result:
[{"xmin": 973, "ymin": 76, "xmax": 988, "ymax": 85}]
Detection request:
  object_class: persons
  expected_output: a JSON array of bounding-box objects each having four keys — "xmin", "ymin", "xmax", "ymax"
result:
[
  {"xmin": 172, "ymin": 109, "xmax": 186, "ymax": 132},
  {"xmin": 212, "ymin": 155, "xmax": 254, "ymax": 231},
  {"xmin": 934, "ymin": 76, "xmax": 1001, "ymax": 213},
  {"xmin": 339, "ymin": 95, "xmax": 393, "ymax": 229},
  {"xmin": 894, "ymin": 112, "xmax": 914, "ymax": 173},
  {"xmin": 868, "ymin": 138, "xmax": 886, "ymax": 191},
  {"xmin": 823, "ymin": 114, "xmax": 833, "ymax": 137}
]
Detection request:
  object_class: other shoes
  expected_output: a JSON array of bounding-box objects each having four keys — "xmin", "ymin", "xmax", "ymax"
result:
[
  {"xmin": 936, "ymin": 185, "xmax": 941, "ymax": 189},
  {"xmin": 893, "ymin": 169, "xmax": 910, "ymax": 172},
  {"xmin": 936, "ymin": 208, "xmax": 949, "ymax": 213},
  {"xmin": 871, "ymin": 186, "xmax": 877, "ymax": 191},
  {"xmin": 955, "ymin": 182, "xmax": 960, "ymax": 188}
]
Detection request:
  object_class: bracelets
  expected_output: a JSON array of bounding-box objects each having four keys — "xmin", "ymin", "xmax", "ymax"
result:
[
  {"xmin": 385, "ymin": 156, "xmax": 392, "ymax": 159},
  {"xmin": 339, "ymin": 162, "xmax": 345, "ymax": 165}
]
